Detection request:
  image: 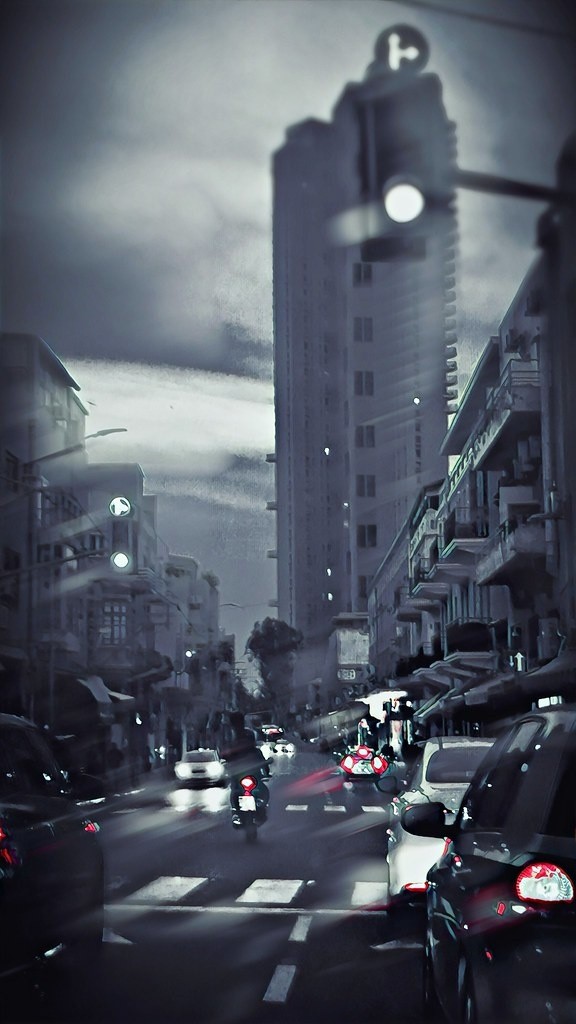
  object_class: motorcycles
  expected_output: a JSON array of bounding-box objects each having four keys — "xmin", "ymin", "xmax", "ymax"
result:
[{"xmin": 223, "ymin": 757, "xmax": 275, "ymax": 842}]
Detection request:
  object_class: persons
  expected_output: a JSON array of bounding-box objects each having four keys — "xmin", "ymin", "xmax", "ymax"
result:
[{"xmin": 224, "ymin": 730, "xmax": 270, "ymax": 829}]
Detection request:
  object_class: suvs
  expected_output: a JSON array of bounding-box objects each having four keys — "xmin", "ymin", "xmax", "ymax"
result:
[{"xmin": 426, "ymin": 703, "xmax": 576, "ymax": 1024}]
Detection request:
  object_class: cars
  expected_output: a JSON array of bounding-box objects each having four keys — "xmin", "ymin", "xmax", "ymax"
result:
[
  {"xmin": 0, "ymin": 715, "xmax": 104, "ymax": 1024},
  {"xmin": 343, "ymin": 747, "xmax": 382, "ymax": 782},
  {"xmin": 174, "ymin": 748, "xmax": 226, "ymax": 789},
  {"xmin": 386, "ymin": 736, "xmax": 497, "ymax": 921},
  {"xmin": 257, "ymin": 725, "xmax": 283, "ymax": 742}
]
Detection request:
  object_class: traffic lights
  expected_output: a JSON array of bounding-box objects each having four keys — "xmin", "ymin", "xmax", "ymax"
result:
[
  {"xmin": 110, "ymin": 551, "xmax": 131, "ymax": 571},
  {"xmin": 109, "ymin": 496, "xmax": 135, "ymax": 517},
  {"xmin": 186, "ymin": 651, "xmax": 192, "ymax": 658},
  {"xmin": 384, "ymin": 183, "xmax": 426, "ymax": 223}
]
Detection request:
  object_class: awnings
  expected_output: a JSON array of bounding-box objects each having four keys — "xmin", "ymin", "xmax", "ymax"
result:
[
  {"xmin": 108, "ymin": 692, "xmax": 134, "ymax": 704},
  {"xmin": 413, "ymin": 658, "xmax": 576, "ymax": 722}
]
